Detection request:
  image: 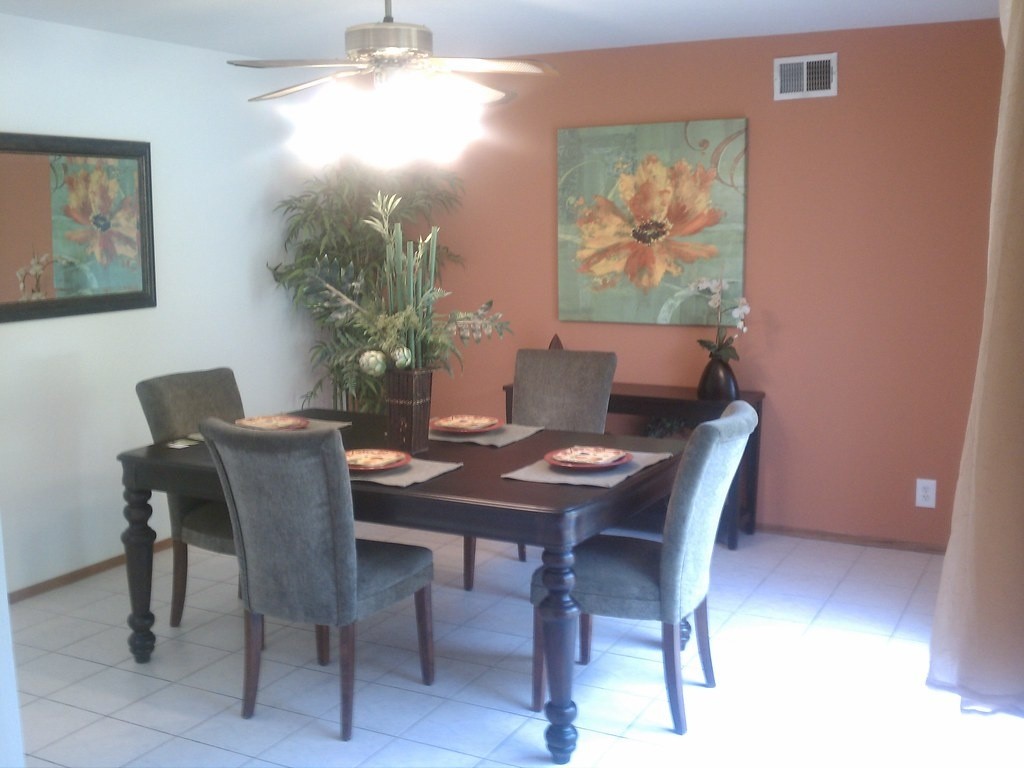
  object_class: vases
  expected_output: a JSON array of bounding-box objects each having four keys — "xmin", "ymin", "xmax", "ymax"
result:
[
  {"xmin": 378, "ymin": 370, "xmax": 434, "ymax": 455},
  {"xmin": 699, "ymin": 358, "xmax": 739, "ymax": 399}
]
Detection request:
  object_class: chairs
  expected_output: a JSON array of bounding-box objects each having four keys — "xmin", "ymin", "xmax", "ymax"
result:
[
  {"xmin": 201, "ymin": 416, "xmax": 435, "ymax": 742},
  {"xmin": 463, "ymin": 347, "xmax": 616, "ymax": 590},
  {"xmin": 531, "ymin": 400, "xmax": 758, "ymax": 735},
  {"xmin": 136, "ymin": 367, "xmax": 266, "ymax": 650}
]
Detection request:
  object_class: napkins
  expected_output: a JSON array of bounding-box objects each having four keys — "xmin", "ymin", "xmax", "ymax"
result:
[
  {"xmin": 501, "ymin": 448, "xmax": 673, "ymax": 489},
  {"xmin": 428, "ymin": 416, "xmax": 547, "ymax": 447},
  {"xmin": 349, "ymin": 456, "xmax": 464, "ymax": 488},
  {"xmin": 187, "ymin": 418, "xmax": 351, "ymax": 442}
]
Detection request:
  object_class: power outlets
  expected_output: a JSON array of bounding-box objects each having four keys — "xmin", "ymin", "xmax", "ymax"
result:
[{"xmin": 915, "ymin": 477, "xmax": 937, "ymax": 509}]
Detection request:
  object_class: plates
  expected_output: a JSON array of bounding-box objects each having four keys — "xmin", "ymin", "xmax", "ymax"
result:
[
  {"xmin": 544, "ymin": 447, "xmax": 633, "ymax": 470},
  {"xmin": 429, "ymin": 415, "xmax": 505, "ymax": 433},
  {"xmin": 345, "ymin": 449, "xmax": 412, "ymax": 471},
  {"xmin": 234, "ymin": 416, "xmax": 309, "ymax": 431}
]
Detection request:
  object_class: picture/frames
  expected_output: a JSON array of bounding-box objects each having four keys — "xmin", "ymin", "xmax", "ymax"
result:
[{"xmin": 0, "ymin": 132, "xmax": 157, "ymax": 324}]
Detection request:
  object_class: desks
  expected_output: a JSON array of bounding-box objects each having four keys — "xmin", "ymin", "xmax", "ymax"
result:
[{"xmin": 502, "ymin": 380, "xmax": 766, "ymax": 551}]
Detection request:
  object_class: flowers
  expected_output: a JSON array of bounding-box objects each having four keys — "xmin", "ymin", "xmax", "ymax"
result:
[
  {"xmin": 694, "ymin": 275, "xmax": 751, "ymax": 363},
  {"xmin": 335, "ymin": 192, "xmax": 465, "ymax": 401}
]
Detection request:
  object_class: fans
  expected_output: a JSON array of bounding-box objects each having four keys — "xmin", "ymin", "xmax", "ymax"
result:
[{"xmin": 228, "ymin": 0, "xmax": 561, "ymax": 105}]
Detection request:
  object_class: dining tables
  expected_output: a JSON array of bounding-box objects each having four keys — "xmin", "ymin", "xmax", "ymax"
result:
[{"xmin": 117, "ymin": 409, "xmax": 691, "ymax": 765}]
